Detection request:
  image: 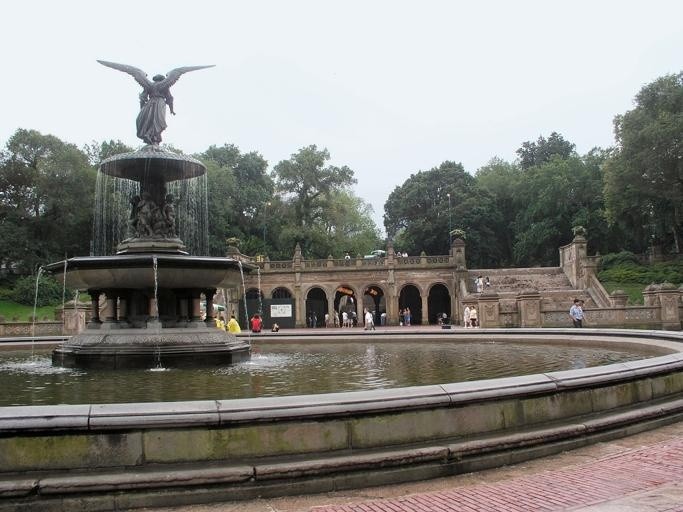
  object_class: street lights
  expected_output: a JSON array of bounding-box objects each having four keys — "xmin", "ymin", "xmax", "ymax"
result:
[
  {"xmin": 264, "ymin": 200, "xmax": 271, "ymax": 262},
  {"xmin": 446, "ymin": 192, "xmax": 452, "ymax": 256}
]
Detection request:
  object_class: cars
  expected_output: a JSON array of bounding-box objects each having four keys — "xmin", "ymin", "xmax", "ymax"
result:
[{"xmin": 363, "ymin": 249, "xmax": 384, "ymax": 258}]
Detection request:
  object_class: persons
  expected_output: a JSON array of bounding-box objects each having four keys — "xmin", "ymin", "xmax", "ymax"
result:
[
  {"xmin": 133, "ymin": 72, "xmax": 176, "ymax": 149},
  {"xmin": 396, "ymin": 251, "xmax": 402, "ymax": 257},
  {"xmin": 462, "ymin": 304, "xmax": 471, "ymax": 327},
  {"xmin": 474, "ymin": 275, "xmax": 485, "ymax": 292},
  {"xmin": 567, "ymin": 298, "xmax": 587, "ymax": 327},
  {"xmin": 401, "ymin": 249, "xmax": 408, "ymax": 258},
  {"xmin": 127, "ymin": 189, "xmax": 178, "ymax": 239},
  {"xmin": 485, "ymin": 276, "xmax": 493, "ymax": 287},
  {"xmin": 344, "ymin": 252, "xmax": 351, "ymax": 259},
  {"xmin": 469, "ymin": 306, "xmax": 478, "ymax": 329},
  {"xmin": 212, "ymin": 307, "xmax": 447, "ymax": 336}
]
